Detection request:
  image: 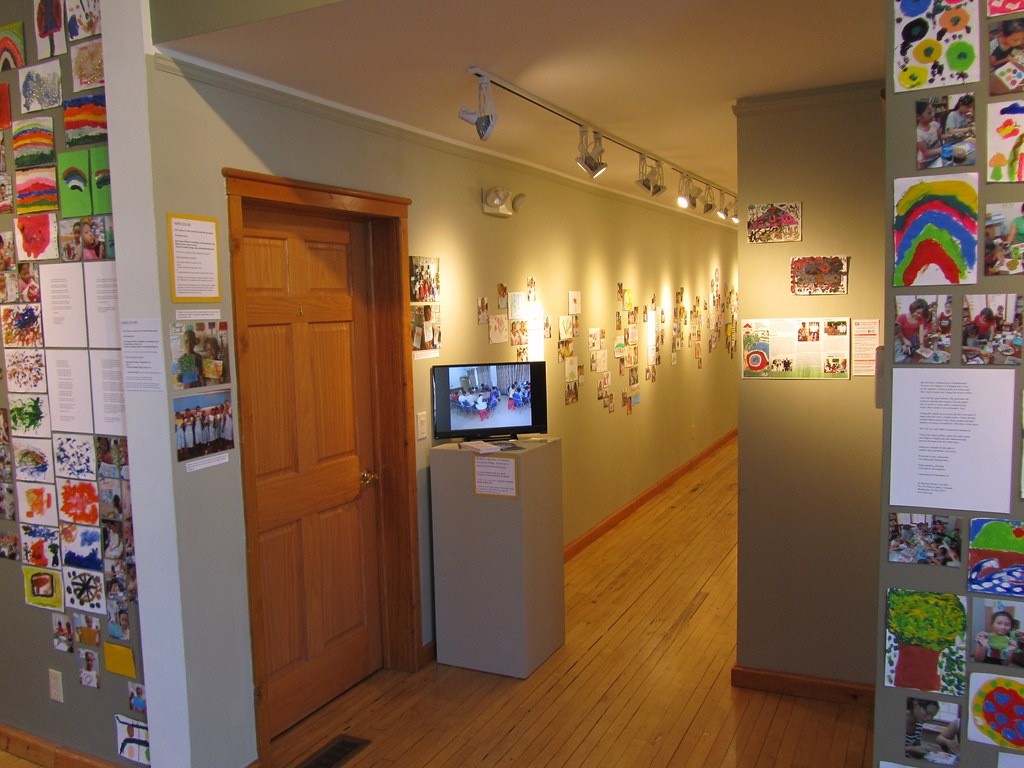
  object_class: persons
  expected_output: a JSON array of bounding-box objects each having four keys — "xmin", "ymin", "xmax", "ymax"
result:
[
  {"xmin": 78, "ymin": 652, "xmax": 101, "ymax": 688},
  {"xmin": 56, "ymin": 620, "xmax": 73, "ymax": 641},
  {"xmin": 782, "ymin": 321, "xmax": 846, "ymax": 374},
  {"xmin": 411, "ymin": 264, "xmax": 437, "ymax": 350},
  {"xmin": 0, "ymin": 235, "xmax": 9, "ymax": 303},
  {"xmin": 63, "ymin": 222, "xmax": 115, "ymax": 261},
  {"xmin": 75, "ymin": 616, "xmax": 101, "ymax": 646},
  {"xmin": 893, "ymin": 296, "xmax": 951, "ymax": 363},
  {"xmin": 96, "ymin": 436, "xmax": 129, "ymax": 478},
  {"xmin": 915, "ymin": 94, "xmax": 974, "ymax": 168},
  {"xmin": 18, "ymin": 263, "xmax": 36, "ymax": 301},
  {"xmin": 451, "ymin": 277, "xmax": 738, "ymax": 415},
  {"xmin": 175, "ymin": 330, "xmax": 234, "ymax": 462},
  {"xmin": 128, "ymin": 687, "xmax": 147, "ymax": 714},
  {"xmin": 888, "ymin": 517, "xmax": 1024, "ymax": 762},
  {"xmin": 990, "ymin": 18, "xmax": 1024, "ymax": 66},
  {"xmin": 0, "ymin": 183, "xmax": 9, "ymax": 202},
  {"xmin": 963, "ymin": 305, "xmax": 1024, "ymax": 360},
  {"xmin": 104, "ymin": 494, "xmax": 137, "ymax": 639},
  {"xmin": 986, "ymin": 203, "xmax": 1024, "ymax": 273}
]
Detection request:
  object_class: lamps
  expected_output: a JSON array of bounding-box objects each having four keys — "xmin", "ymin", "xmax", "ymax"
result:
[
  {"xmin": 700, "ymin": 185, "xmax": 716, "ymax": 214},
  {"xmin": 732, "ymin": 197, "xmax": 738, "ymax": 222},
  {"xmin": 717, "ymin": 192, "xmax": 733, "ymax": 220},
  {"xmin": 456, "ymin": 74, "xmax": 498, "ymax": 142},
  {"xmin": 635, "ymin": 153, "xmax": 667, "ymax": 197},
  {"xmin": 575, "ymin": 124, "xmax": 608, "ymax": 176},
  {"xmin": 676, "ymin": 172, "xmax": 702, "ymax": 209}
]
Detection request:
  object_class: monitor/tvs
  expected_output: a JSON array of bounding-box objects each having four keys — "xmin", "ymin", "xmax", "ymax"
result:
[{"xmin": 433, "ymin": 361, "xmax": 547, "ymax": 442}]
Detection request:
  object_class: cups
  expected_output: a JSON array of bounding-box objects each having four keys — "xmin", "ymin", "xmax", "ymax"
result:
[{"xmin": 941, "ymin": 144, "xmax": 954, "ymax": 167}]
